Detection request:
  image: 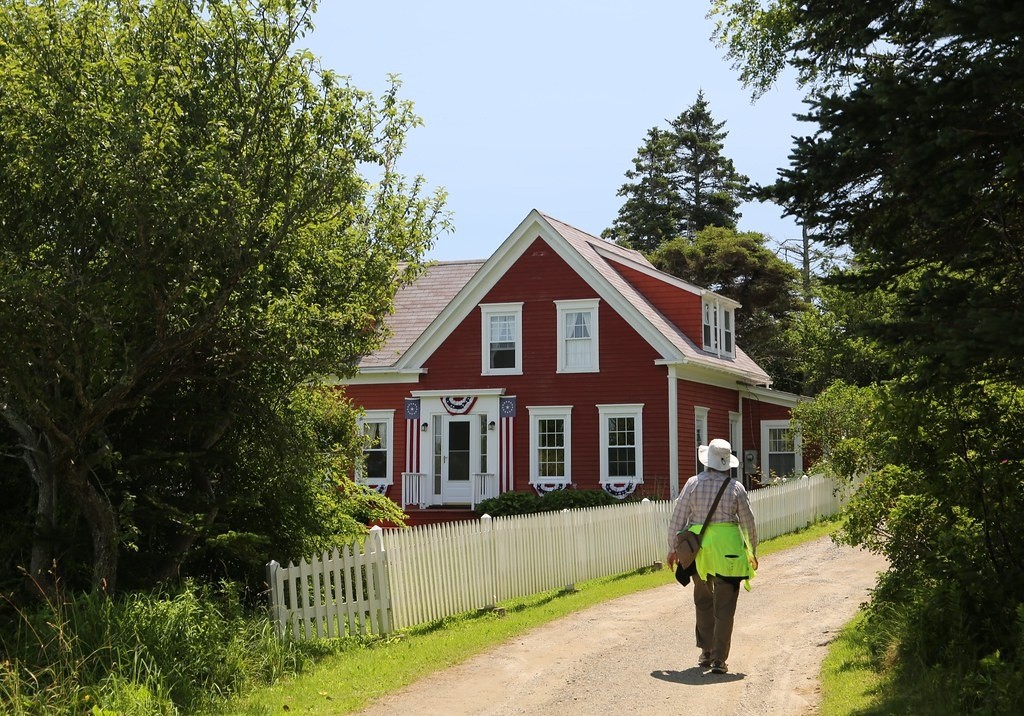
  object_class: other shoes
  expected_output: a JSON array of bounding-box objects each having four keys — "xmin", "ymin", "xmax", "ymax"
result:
[
  {"xmin": 697, "ymin": 652, "xmax": 712, "ymax": 665},
  {"xmin": 710, "ymin": 659, "xmax": 728, "ymax": 673}
]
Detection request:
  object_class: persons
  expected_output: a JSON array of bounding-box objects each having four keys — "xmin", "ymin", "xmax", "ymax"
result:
[{"xmin": 666, "ymin": 439, "xmax": 759, "ymax": 674}]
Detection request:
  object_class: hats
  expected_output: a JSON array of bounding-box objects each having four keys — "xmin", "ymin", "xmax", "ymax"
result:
[{"xmin": 698, "ymin": 439, "xmax": 740, "ymax": 471}]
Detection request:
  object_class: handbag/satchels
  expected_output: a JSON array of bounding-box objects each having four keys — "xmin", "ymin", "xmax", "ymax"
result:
[{"xmin": 672, "ymin": 531, "xmax": 701, "ymax": 570}]
